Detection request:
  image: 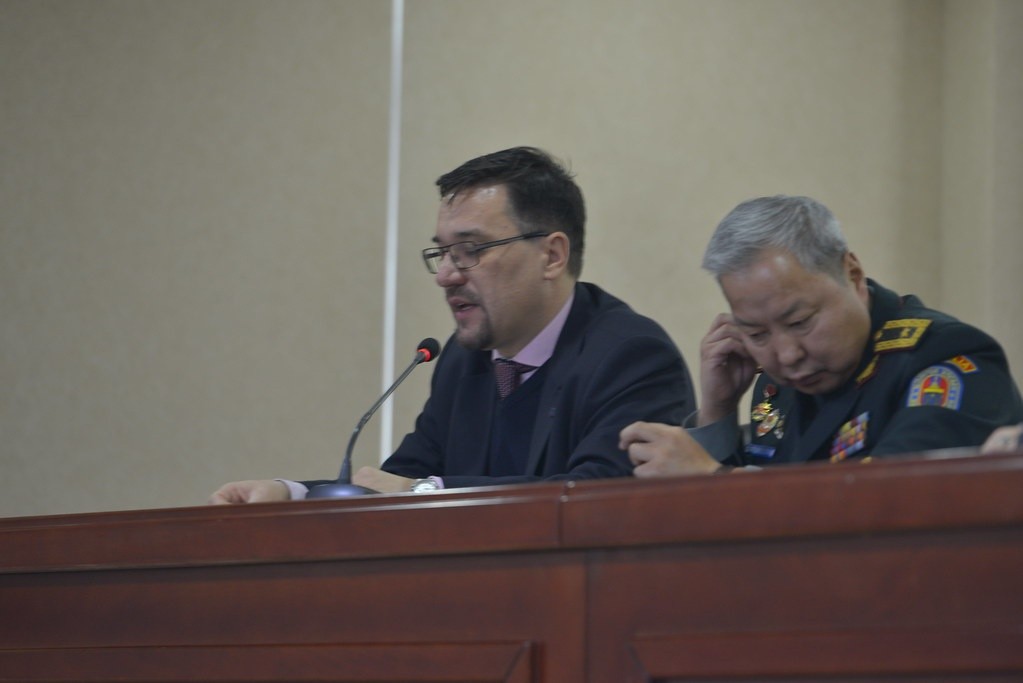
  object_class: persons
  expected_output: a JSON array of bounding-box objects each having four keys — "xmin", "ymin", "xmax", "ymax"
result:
[
  {"xmin": 620, "ymin": 195, "xmax": 1023, "ymax": 478},
  {"xmin": 212, "ymin": 146, "xmax": 697, "ymax": 503}
]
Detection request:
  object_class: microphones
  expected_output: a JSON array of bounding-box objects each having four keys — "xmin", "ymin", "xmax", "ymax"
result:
[{"xmin": 305, "ymin": 338, "xmax": 440, "ymax": 500}]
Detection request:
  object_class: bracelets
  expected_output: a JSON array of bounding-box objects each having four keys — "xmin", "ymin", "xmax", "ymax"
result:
[{"xmin": 714, "ymin": 466, "xmax": 735, "ymax": 474}]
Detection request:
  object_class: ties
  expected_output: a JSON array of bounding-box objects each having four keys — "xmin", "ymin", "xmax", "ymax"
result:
[{"xmin": 494, "ymin": 360, "xmax": 518, "ymax": 401}]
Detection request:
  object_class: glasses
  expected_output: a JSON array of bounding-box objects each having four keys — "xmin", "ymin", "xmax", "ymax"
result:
[{"xmin": 422, "ymin": 232, "xmax": 551, "ymax": 274}]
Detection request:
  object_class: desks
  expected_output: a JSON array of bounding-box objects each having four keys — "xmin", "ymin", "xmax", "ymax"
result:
[{"xmin": 0, "ymin": 449, "xmax": 1023, "ymax": 683}]
